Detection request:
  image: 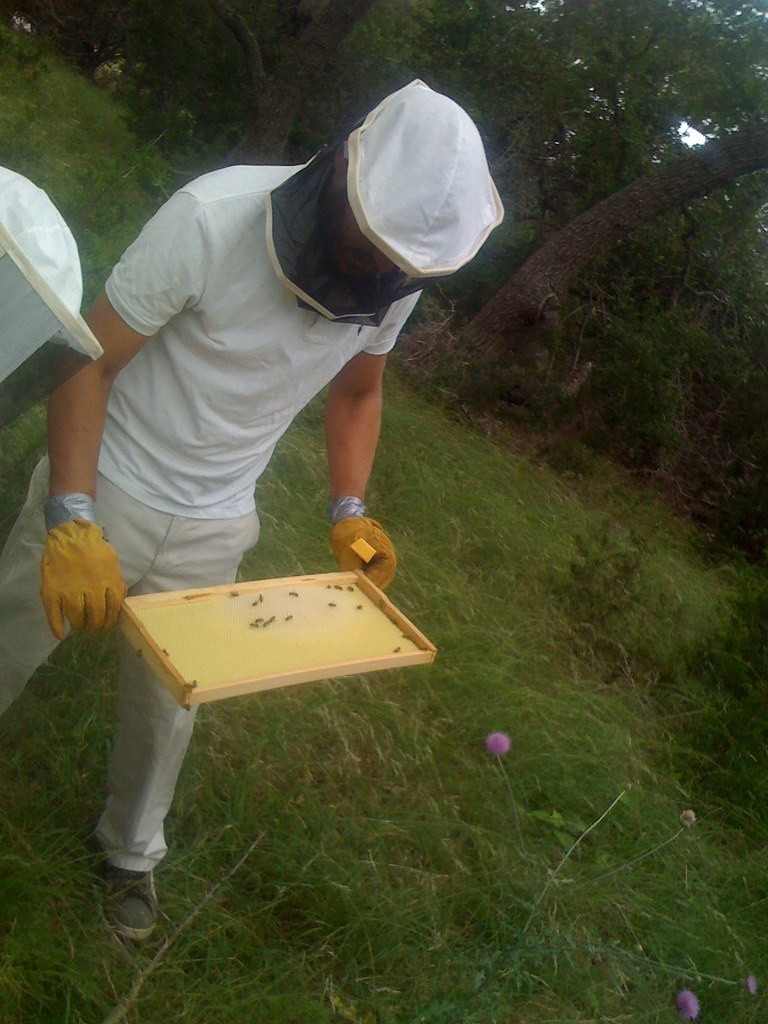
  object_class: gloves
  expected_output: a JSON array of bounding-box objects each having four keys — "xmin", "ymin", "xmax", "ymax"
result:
[
  {"xmin": 41, "ymin": 492, "xmax": 128, "ymax": 640},
  {"xmin": 331, "ymin": 494, "xmax": 397, "ymax": 590}
]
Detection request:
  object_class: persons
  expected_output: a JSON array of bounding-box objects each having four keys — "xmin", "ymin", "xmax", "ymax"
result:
[{"xmin": 0, "ymin": 78, "xmax": 504, "ymax": 939}]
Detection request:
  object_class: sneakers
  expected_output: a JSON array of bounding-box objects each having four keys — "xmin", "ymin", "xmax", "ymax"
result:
[{"xmin": 104, "ymin": 862, "xmax": 157, "ymax": 939}]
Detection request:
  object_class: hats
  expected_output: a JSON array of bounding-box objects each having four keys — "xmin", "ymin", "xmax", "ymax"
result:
[
  {"xmin": 347, "ymin": 78, "xmax": 506, "ymax": 280},
  {"xmin": 0, "ymin": 165, "xmax": 105, "ymax": 362}
]
